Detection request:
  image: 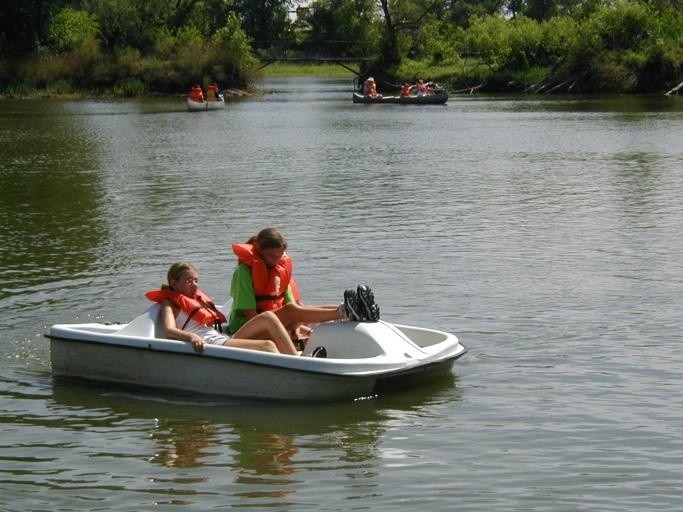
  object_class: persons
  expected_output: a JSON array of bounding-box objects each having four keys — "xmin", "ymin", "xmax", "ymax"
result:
[
  {"xmin": 159, "ymin": 260, "xmax": 327, "ymax": 360},
  {"xmin": 224, "ymin": 226, "xmax": 381, "ymax": 343},
  {"xmin": 398, "ymin": 79, "xmax": 435, "ymax": 99},
  {"xmin": 206, "ymin": 83, "xmax": 221, "ymax": 101},
  {"xmin": 187, "ymin": 83, "xmax": 204, "ymax": 103},
  {"xmin": 363, "ymin": 76, "xmax": 382, "ymax": 100}
]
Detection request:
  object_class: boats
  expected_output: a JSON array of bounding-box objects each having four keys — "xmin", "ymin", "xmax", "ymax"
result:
[
  {"xmin": 186, "ymin": 92, "xmax": 225, "ymax": 112},
  {"xmin": 351, "ymin": 87, "xmax": 449, "ymax": 106},
  {"xmin": 42, "ymin": 299, "xmax": 471, "ymax": 406}
]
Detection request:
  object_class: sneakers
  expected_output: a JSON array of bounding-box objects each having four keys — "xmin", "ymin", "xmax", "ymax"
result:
[
  {"xmin": 311, "ymin": 346, "xmax": 327, "ymax": 357},
  {"xmin": 338, "ymin": 283, "xmax": 379, "ymax": 322}
]
